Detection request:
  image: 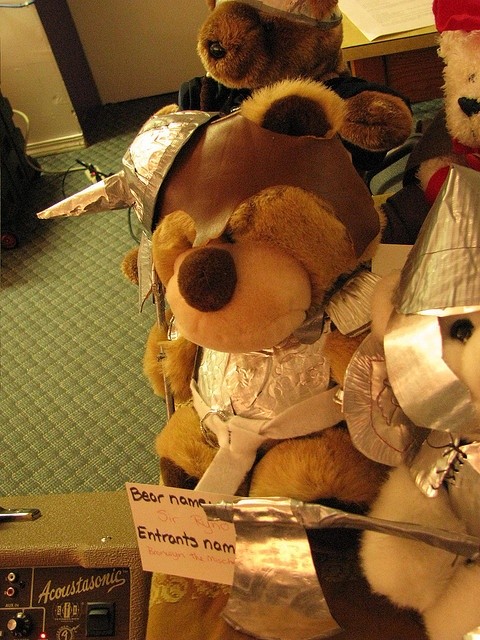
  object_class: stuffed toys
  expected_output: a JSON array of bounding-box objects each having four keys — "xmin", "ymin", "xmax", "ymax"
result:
[
  {"xmin": 36, "ymin": 79, "xmax": 385, "ymax": 540},
  {"xmin": 416, "ymin": 0, "xmax": 480, "ymax": 202},
  {"xmin": 360, "ymin": 164, "xmax": 479, "ymax": 640},
  {"xmin": 179, "ymin": 0, "xmax": 415, "ymax": 176}
]
